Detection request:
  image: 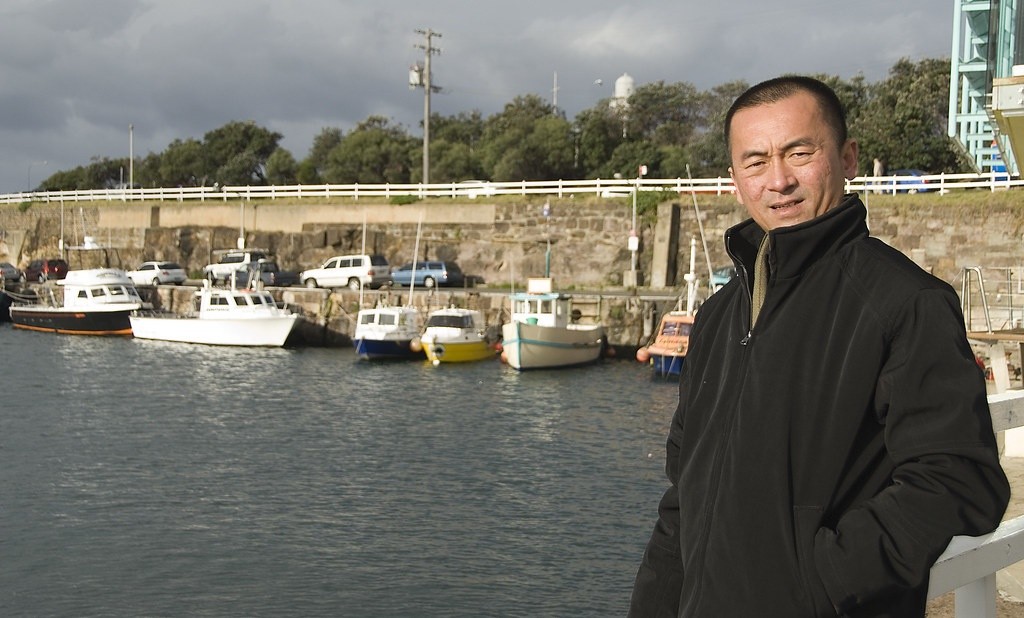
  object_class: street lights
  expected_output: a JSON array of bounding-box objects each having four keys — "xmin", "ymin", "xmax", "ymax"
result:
[
  {"xmin": 29, "ymin": 162, "xmax": 48, "ymax": 192},
  {"xmin": 129, "ymin": 125, "xmax": 134, "ymax": 191}
]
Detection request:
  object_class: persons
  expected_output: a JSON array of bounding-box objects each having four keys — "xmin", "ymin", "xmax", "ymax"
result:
[
  {"xmin": 192, "ymin": 174, "xmax": 198, "ymax": 187},
  {"xmin": 628, "ymin": 76, "xmax": 1011, "ymax": 618},
  {"xmin": 641, "ymin": 160, "xmax": 650, "ymax": 186},
  {"xmin": 872, "ymin": 157, "xmax": 884, "ymax": 195}
]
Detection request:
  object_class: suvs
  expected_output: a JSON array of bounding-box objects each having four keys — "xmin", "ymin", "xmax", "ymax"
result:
[
  {"xmin": 21, "ymin": 259, "xmax": 69, "ymax": 283},
  {"xmin": 299, "ymin": 255, "xmax": 391, "ymax": 290},
  {"xmin": 389, "ymin": 261, "xmax": 457, "ymax": 287},
  {"xmin": 203, "ymin": 251, "xmax": 273, "ymax": 285}
]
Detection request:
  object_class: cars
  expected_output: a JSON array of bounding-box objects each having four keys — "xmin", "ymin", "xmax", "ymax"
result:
[
  {"xmin": 129, "ymin": 259, "xmax": 186, "ymax": 286},
  {"xmin": 863, "ymin": 170, "xmax": 933, "ymax": 194},
  {"xmin": 454, "ymin": 180, "xmax": 494, "ymax": 199},
  {"xmin": 709, "ymin": 263, "xmax": 737, "ymax": 292},
  {"xmin": 0, "ymin": 263, "xmax": 20, "ymax": 280}
]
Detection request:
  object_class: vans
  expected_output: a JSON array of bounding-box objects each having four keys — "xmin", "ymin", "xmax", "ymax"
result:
[{"xmin": 114, "ymin": 183, "xmax": 142, "ymax": 190}]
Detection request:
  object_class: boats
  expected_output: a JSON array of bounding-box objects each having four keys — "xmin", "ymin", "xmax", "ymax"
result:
[
  {"xmin": 498, "ymin": 291, "xmax": 605, "ymax": 370},
  {"xmin": 7, "ymin": 267, "xmax": 151, "ymax": 336},
  {"xmin": 351, "ymin": 305, "xmax": 425, "ymax": 360},
  {"xmin": 129, "ymin": 263, "xmax": 300, "ymax": 349},
  {"xmin": 647, "ymin": 273, "xmax": 698, "ymax": 377},
  {"xmin": 421, "ymin": 308, "xmax": 500, "ymax": 363}
]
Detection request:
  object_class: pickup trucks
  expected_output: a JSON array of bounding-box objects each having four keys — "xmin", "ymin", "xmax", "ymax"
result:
[{"xmin": 226, "ymin": 262, "xmax": 298, "ymax": 287}]
